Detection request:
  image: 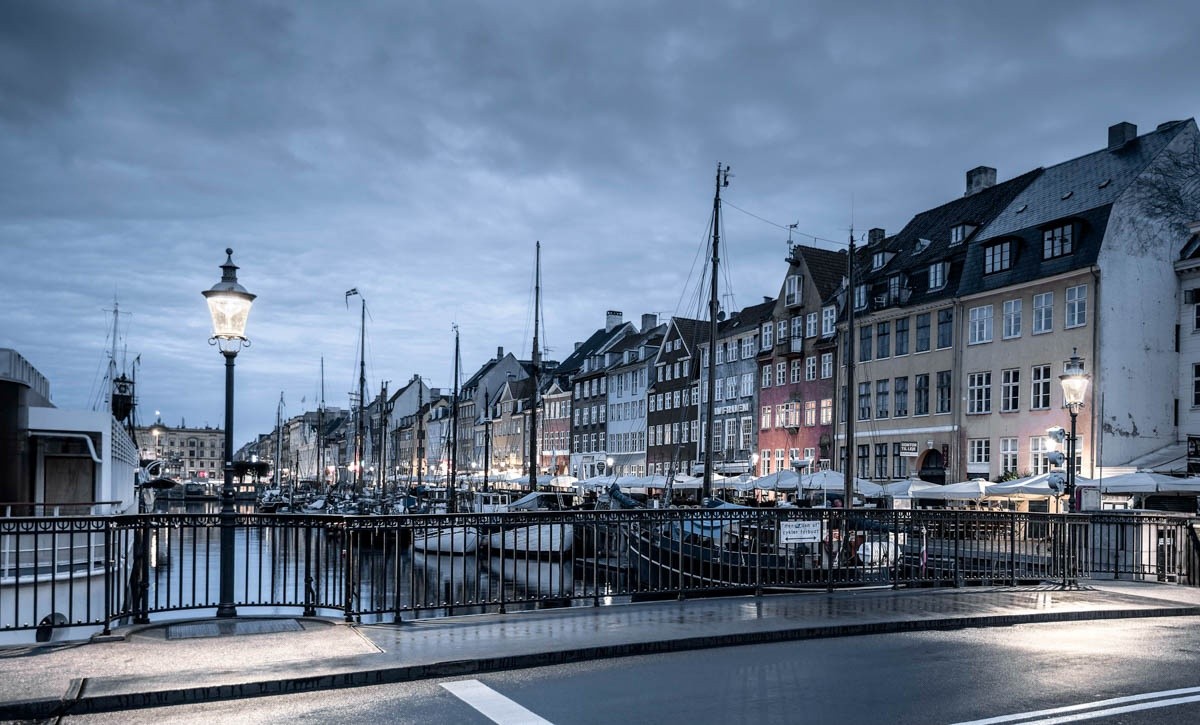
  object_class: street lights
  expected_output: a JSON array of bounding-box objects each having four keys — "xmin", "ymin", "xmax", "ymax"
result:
[
  {"xmin": 202, "ymin": 248, "xmax": 258, "ymax": 619},
  {"xmin": 151, "ymin": 429, "xmax": 159, "ymax": 462},
  {"xmin": 1058, "ymin": 347, "xmax": 1092, "ymax": 578}
]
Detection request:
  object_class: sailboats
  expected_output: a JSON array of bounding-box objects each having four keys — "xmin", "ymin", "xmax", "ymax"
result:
[{"xmin": 259, "ymin": 161, "xmax": 913, "ymax": 595}]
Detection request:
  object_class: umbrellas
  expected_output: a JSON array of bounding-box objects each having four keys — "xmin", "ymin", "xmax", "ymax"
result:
[{"xmin": 383, "ymin": 469, "xmax": 1200, "ymax": 510}]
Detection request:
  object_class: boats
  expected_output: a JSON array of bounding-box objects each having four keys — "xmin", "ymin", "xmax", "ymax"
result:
[{"xmin": 156, "ymin": 481, "xmax": 267, "ymax": 502}]
